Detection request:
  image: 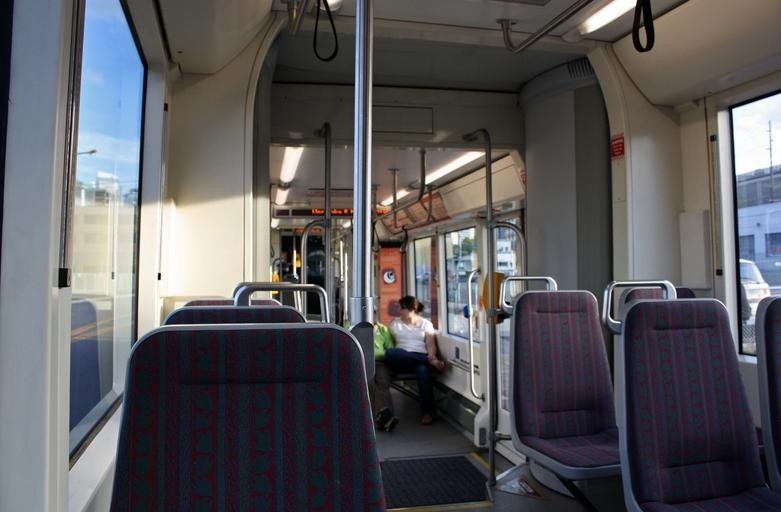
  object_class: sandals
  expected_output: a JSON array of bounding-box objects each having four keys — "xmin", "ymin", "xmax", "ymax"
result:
[
  {"xmin": 436, "ymin": 359, "xmax": 446, "ymax": 369},
  {"xmin": 422, "ymin": 415, "xmax": 432, "ymax": 424}
]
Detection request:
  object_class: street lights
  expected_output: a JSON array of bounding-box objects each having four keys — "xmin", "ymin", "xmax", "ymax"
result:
[{"xmin": 75, "ymin": 147, "xmax": 96, "ymax": 159}]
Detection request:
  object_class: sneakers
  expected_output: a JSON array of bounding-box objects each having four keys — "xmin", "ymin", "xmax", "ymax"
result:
[{"xmin": 380, "ymin": 416, "xmax": 399, "ymax": 432}]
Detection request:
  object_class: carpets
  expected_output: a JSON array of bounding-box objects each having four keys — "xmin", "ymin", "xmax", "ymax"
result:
[{"xmin": 376, "ymin": 451, "xmax": 498, "ymax": 511}]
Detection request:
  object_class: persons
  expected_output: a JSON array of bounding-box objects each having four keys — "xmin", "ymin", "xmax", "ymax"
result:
[
  {"xmin": 344, "ymin": 305, "xmax": 397, "ymax": 432},
  {"xmin": 383, "ymin": 295, "xmax": 445, "ymax": 425}
]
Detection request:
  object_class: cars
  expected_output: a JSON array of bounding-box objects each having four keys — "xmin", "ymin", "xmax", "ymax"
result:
[{"xmin": 740, "ymin": 260, "xmax": 772, "ymax": 323}]
